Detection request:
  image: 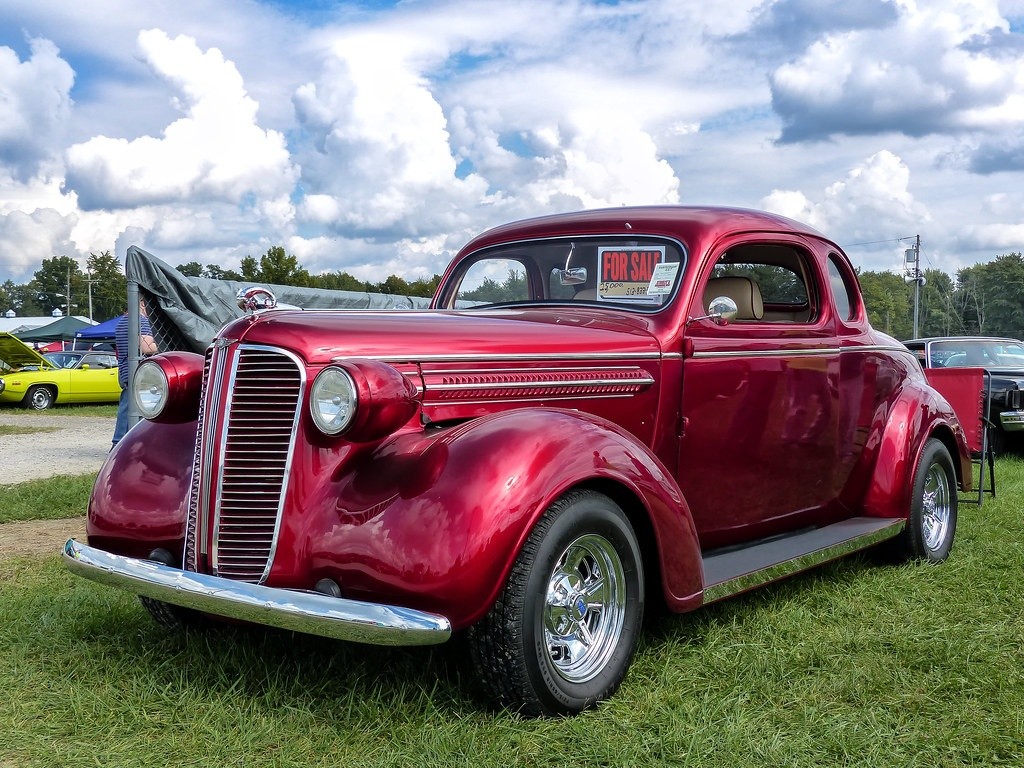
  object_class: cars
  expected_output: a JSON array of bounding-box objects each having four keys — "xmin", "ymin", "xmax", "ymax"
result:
[
  {"xmin": 901, "ymin": 336, "xmax": 1024, "ymax": 460},
  {"xmin": 0, "ymin": 331, "xmax": 124, "ymax": 411},
  {"xmin": 60, "ymin": 203, "xmax": 975, "ymax": 720}
]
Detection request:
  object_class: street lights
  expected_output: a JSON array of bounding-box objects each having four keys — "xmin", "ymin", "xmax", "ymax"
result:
[{"xmin": 56, "ymin": 293, "xmax": 70, "ymax": 317}]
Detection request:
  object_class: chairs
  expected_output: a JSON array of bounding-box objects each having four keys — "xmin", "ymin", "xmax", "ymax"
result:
[
  {"xmin": 703, "ymin": 276, "xmax": 763, "ymax": 322},
  {"xmin": 922, "ymin": 368, "xmax": 996, "ymax": 507}
]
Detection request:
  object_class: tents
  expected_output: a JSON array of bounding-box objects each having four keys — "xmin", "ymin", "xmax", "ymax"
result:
[{"xmin": 0, "ymin": 308, "xmax": 124, "ymax": 367}]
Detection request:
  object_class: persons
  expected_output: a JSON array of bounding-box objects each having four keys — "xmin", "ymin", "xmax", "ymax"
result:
[{"xmin": 109, "ymin": 296, "xmax": 158, "ymax": 453}]
